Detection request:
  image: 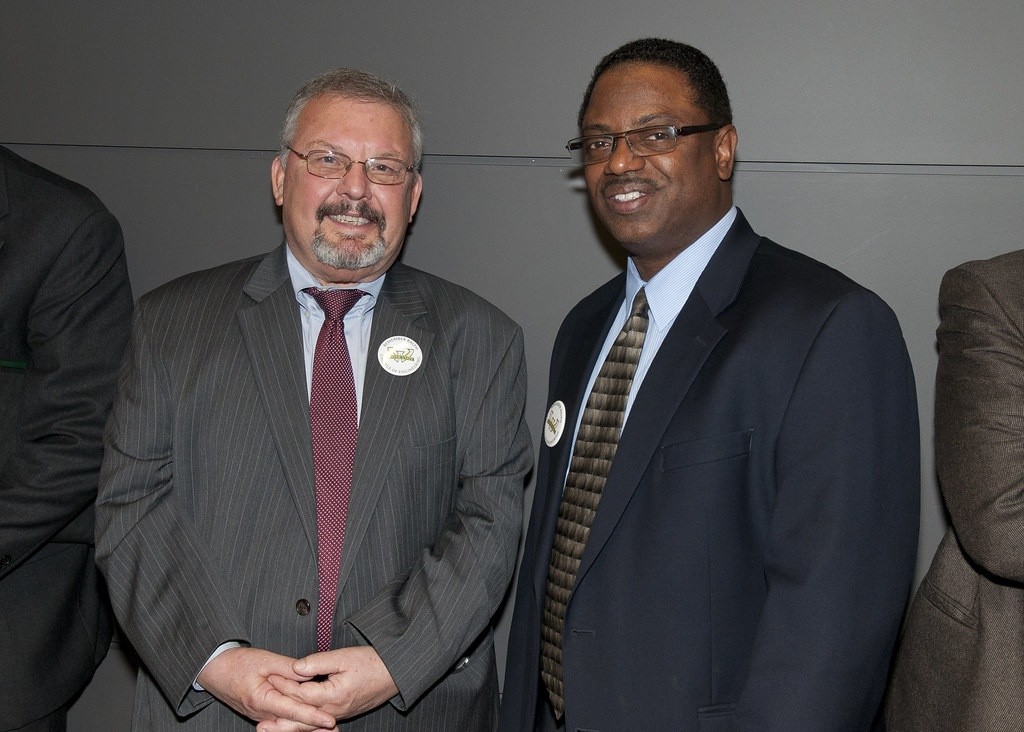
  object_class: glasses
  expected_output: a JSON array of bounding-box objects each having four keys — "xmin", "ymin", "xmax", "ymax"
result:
[
  {"xmin": 286, "ymin": 146, "xmax": 414, "ymax": 186},
  {"xmin": 565, "ymin": 122, "xmax": 728, "ymax": 167}
]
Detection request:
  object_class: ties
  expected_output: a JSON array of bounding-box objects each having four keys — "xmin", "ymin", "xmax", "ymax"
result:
[
  {"xmin": 543, "ymin": 290, "xmax": 650, "ymax": 720},
  {"xmin": 302, "ymin": 286, "xmax": 371, "ymax": 655}
]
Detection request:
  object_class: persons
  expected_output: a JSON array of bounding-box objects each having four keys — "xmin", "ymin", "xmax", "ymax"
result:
[
  {"xmin": 496, "ymin": 39, "xmax": 921, "ymax": 732},
  {"xmin": 93, "ymin": 70, "xmax": 534, "ymax": 732},
  {"xmin": 883, "ymin": 247, "xmax": 1023, "ymax": 731},
  {"xmin": 0, "ymin": 144, "xmax": 137, "ymax": 732}
]
